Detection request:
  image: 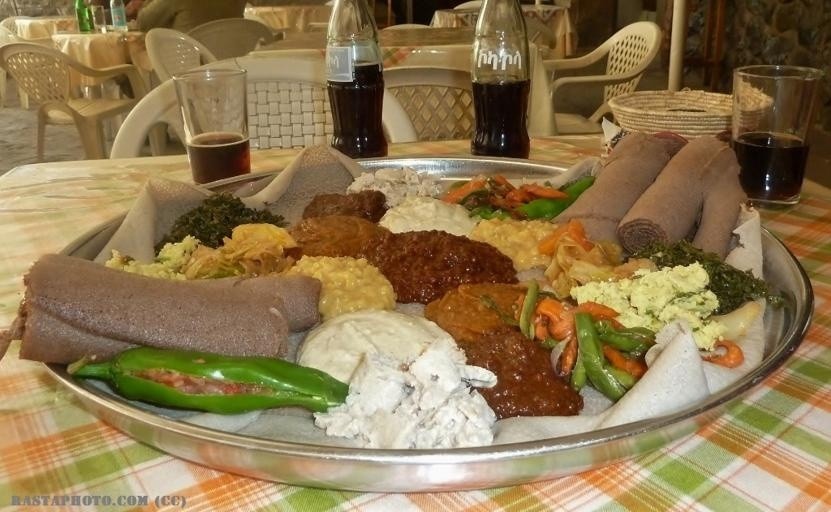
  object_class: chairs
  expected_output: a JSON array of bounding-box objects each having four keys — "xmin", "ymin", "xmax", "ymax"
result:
[{"xmin": 1, "ymin": 0, "xmax": 663, "ymax": 162}]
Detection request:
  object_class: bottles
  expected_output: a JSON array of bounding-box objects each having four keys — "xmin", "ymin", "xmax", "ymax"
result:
[
  {"xmin": 326, "ymin": 0, "xmax": 388, "ymax": 159},
  {"xmin": 76, "ymin": 0, "xmax": 128, "ymax": 34},
  {"xmin": 471, "ymin": 0, "xmax": 531, "ymax": 156}
]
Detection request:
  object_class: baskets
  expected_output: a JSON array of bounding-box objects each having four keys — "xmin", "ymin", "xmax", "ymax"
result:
[
  {"xmin": 607, "ymin": 88, "xmax": 732, "ymax": 143},
  {"xmin": 732, "ymin": 77, "xmax": 774, "ymax": 143}
]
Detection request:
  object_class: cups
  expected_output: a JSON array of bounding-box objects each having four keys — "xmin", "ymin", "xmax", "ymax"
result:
[
  {"xmin": 731, "ymin": 66, "xmax": 826, "ymax": 212},
  {"xmin": 172, "ymin": 70, "xmax": 252, "ymax": 183}
]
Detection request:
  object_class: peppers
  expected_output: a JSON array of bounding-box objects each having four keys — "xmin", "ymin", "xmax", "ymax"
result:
[
  {"xmin": 67, "ymin": 345, "xmax": 350, "ymax": 415},
  {"xmin": 516, "ymin": 174, "xmax": 595, "ymax": 219}
]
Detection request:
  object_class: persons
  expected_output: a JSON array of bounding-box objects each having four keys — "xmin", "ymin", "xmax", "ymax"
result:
[{"xmin": 127, "ymin": 0, "xmax": 244, "ymax": 32}]
